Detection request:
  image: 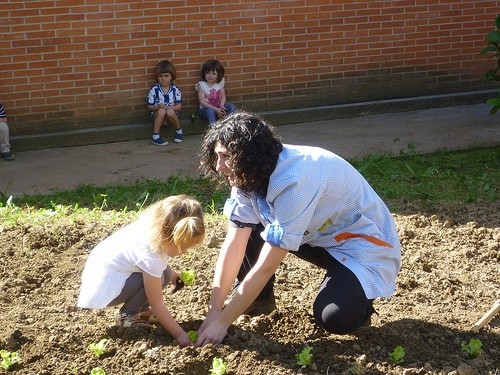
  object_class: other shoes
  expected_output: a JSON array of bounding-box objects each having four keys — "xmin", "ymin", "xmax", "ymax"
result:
[
  {"xmin": 114, "ymin": 308, "xmax": 157, "ymax": 330},
  {"xmin": 223, "ymin": 294, "xmax": 279, "ymax": 315},
  {"xmin": 353, "ymin": 319, "xmax": 371, "ymax": 337},
  {"xmin": 1, "ymin": 152, "xmax": 15, "ymax": 161}
]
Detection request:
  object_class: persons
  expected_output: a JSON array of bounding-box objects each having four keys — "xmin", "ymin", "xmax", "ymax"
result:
[
  {"xmin": 0, "ymin": 104, "xmax": 15, "ymax": 160},
  {"xmin": 146, "ymin": 60, "xmax": 183, "ymax": 145},
  {"xmin": 194, "ymin": 110, "xmax": 402, "ymax": 348},
  {"xmin": 77, "ymin": 194, "xmax": 206, "ymax": 346},
  {"xmin": 195, "ymin": 60, "xmax": 236, "ymax": 125}
]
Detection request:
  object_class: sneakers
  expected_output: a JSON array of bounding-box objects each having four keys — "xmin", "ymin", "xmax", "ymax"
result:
[
  {"xmin": 152, "ymin": 138, "xmax": 168, "ymax": 145},
  {"xmin": 174, "ymin": 132, "xmax": 183, "ymax": 143}
]
